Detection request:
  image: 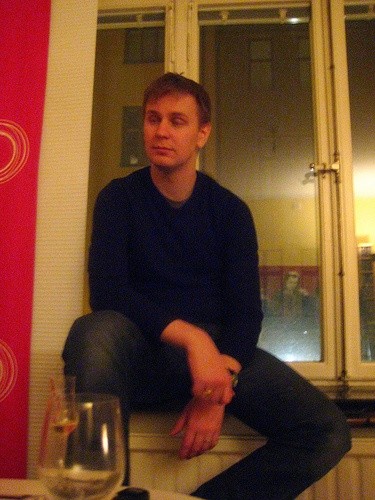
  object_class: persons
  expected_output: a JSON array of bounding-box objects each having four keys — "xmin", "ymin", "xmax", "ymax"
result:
[
  {"xmin": 284, "ymin": 270, "xmax": 301, "ymax": 292},
  {"xmin": 61, "ymin": 71, "xmax": 353, "ymax": 500}
]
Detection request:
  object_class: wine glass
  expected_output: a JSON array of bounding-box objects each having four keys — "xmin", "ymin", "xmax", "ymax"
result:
[{"xmin": 37, "ymin": 393, "xmax": 124, "ymax": 500}]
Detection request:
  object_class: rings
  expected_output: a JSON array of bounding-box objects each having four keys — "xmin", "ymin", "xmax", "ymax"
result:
[{"xmin": 203, "ymin": 387, "xmax": 213, "ymax": 396}]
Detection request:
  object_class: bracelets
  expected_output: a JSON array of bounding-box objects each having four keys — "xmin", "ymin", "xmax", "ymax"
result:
[{"xmin": 226, "ymin": 366, "xmax": 239, "ymax": 388}]
